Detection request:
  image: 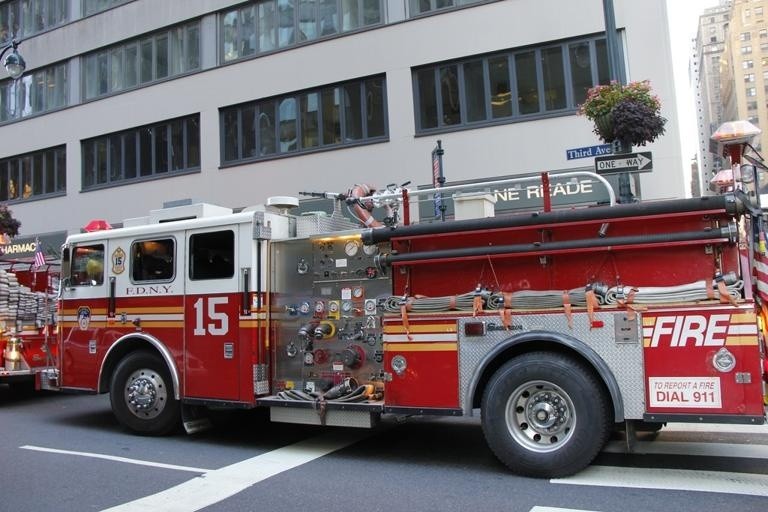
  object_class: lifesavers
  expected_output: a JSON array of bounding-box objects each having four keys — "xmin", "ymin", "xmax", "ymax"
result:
[{"xmin": 352, "ymin": 184, "xmax": 396, "ymax": 227}]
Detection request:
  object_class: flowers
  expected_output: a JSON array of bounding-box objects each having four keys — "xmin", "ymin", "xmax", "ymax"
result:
[
  {"xmin": 0, "ymin": 203, "xmax": 21, "ymax": 240},
  {"xmin": 574, "ymin": 79, "xmax": 668, "ymax": 148}
]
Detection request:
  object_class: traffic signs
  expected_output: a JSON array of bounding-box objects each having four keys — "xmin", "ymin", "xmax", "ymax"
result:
[{"xmin": 595, "ymin": 151, "xmax": 652, "ymax": 175}]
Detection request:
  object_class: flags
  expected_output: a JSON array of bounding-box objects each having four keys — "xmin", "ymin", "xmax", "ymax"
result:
[{"xmin": 33, "ymin": 240, "xmax": 46, "ymax": 268}]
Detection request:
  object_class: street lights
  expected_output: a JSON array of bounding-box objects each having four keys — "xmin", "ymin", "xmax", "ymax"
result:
[{"xmin": 0, "ymin": 36, "xmax": 28, "ymax": 80}]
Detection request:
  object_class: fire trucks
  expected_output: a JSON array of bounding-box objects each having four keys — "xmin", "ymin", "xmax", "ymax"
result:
[
  {"xmin": 39, "ymin": 119, "xmax": 766, "ymax": 481},
  {"xmin": 0, "ymin": 258, "xmax": 59, "ymax": 391}
]
